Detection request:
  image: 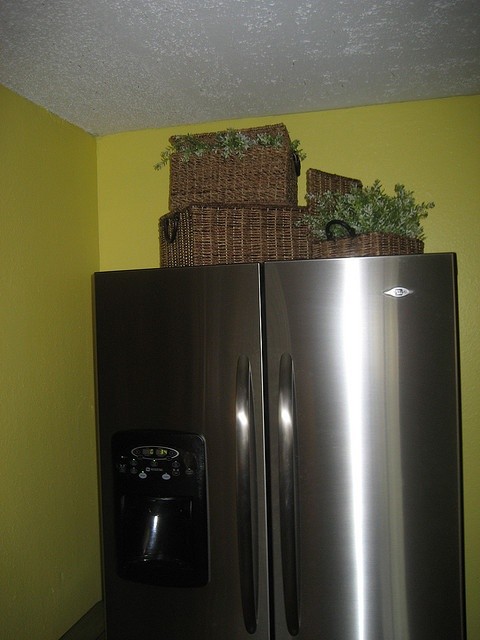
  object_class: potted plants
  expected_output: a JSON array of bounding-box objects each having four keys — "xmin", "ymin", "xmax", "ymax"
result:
[
  {"xmin": 294, "ymin": 167, "xmax": 437, "ymax": 260},
  {"xmin": 153, "ymin": 121, "xmax": 308, "ymax": 209}
]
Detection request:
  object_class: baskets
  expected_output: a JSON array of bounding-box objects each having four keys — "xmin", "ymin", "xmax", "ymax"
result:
[
  {"xmin": 305, "ymin": 167, "xmax": 424, "ymax": 259},
  {"xmin": 158, "ymin": 207, "xmax": 310, "ymax": 268},
  {"xmin": 167, "ymin": 123, "xmax": 301, "ymax": 206}
]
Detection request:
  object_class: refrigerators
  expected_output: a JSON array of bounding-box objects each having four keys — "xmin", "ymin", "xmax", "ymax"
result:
[{"xmin": 93, "ymin": 252, "xmax": 464, "ymax": 640}]
